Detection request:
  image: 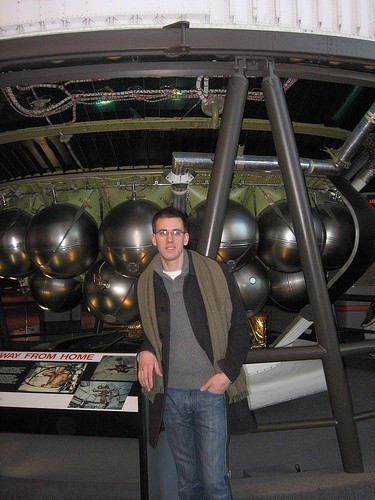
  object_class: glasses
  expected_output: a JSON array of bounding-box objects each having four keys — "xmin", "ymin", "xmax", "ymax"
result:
[{"xmin": 156, "ymin": 229, "xmax": 187, "ymax": 237}]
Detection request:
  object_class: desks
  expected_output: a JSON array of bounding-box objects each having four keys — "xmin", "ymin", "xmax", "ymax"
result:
[{"xmin": 0, "ymin": 352, "xmax": 150, "ymax": 500}]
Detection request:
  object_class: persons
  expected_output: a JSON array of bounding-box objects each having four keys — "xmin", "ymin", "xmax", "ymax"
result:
[{"xmin": 135, "ymin": 206, "xmax": 249, "ymax": 500}]
